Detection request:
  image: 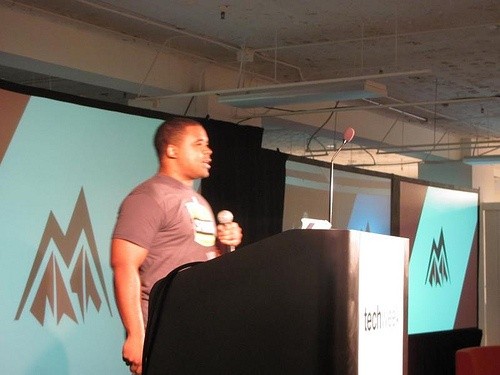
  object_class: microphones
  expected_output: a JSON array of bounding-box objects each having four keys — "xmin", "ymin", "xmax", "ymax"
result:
[
  {"xmin": 329, "ymin": 128, "xmax": 355, "ymax": 223},
  {"xmin": 217, "ymin": 209, "xmax": 236, "ymax": 256}
]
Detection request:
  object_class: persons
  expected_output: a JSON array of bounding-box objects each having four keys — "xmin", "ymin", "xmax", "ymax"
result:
[{"xmin": 109, "ymin": 118, "xmax": 242, "ymax": 375}]
